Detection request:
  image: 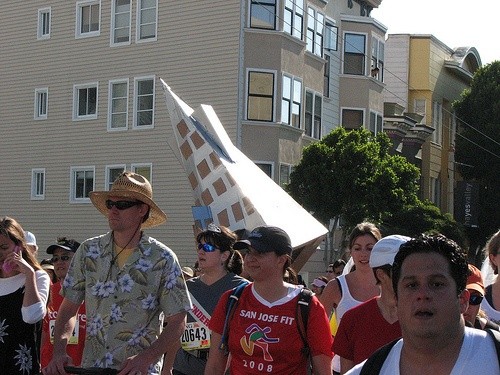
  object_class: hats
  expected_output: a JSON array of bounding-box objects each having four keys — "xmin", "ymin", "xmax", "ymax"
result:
[
  {"xmin": 46, "ymin": 239, "xmax": 77, "ymax": 254},
  {"xmin": 87, "ymin": 172, "xmax": 167, "ymax": 229},
  {"xmin": 181, "ymin": 267, "xmax": 194, "ymax": 278},
  {"xmin": 465, "ymin": 264, "xmax": 485, "ymax": 296},
  {"xmin": 369, "ymin": 235, "xmax": 415, "ymax": 268},
  {"xmin": 311, "ymin": 279, "xmax": 326, "ymax": 287},
  {"xmin": 23, "ymin": 231, "xmax": 38, "ymax": 251},
  {"xmin": 233, "ymin": 226, "xmax": 292, "ymax": 255}
]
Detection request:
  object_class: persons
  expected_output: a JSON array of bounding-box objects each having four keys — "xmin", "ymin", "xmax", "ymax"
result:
[
  {"xmin": 464, "ymin": 230, "xmax": 500, "ymax": 332},
  {"xmin": 0, "ymin": 216, "xmax": 50, "ymax": 375},
  {"xmin": 42, "ymin": 171, "xmax": 193, "ymax": 375},
  {"xmin": 23, "ymin": 230, "xmax": 86, "ymax": 375},
  {"xmin": 163, "ymin": 225, "xmax": 254, "ymax": 375},
  {"xmin": 343, "ymin": 234, "xmax": 500, "ymax": 375},
  {"xmin": 204, "ymin": 226, "xmax": 334, "ymax": 375},
  {"xmin": 371, "ymin": 68, "xmax": 379, "ymax": 77},
  {"xmin": 288, "ymin": 223, "xmax": 413, "ymax": 375}
]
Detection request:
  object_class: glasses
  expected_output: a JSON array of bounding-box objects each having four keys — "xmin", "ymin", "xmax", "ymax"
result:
[
  {"xmin": 470, "ymin": 293, "xmax": 483, "ymax": 305},
  {"xmin": 197, "ymin": 243, "xmax": 223, "ymax": 253},
  {"xmin": 104, "ymin": 199, "xmax": 146, "ymax": 210},
  {"xmin": 325, "ymin": 270, "xmax": 334, "ymax": 273},
  {"xmin": 51, "ymin": 255, "xmax": 75, "ymax": 264}
]
